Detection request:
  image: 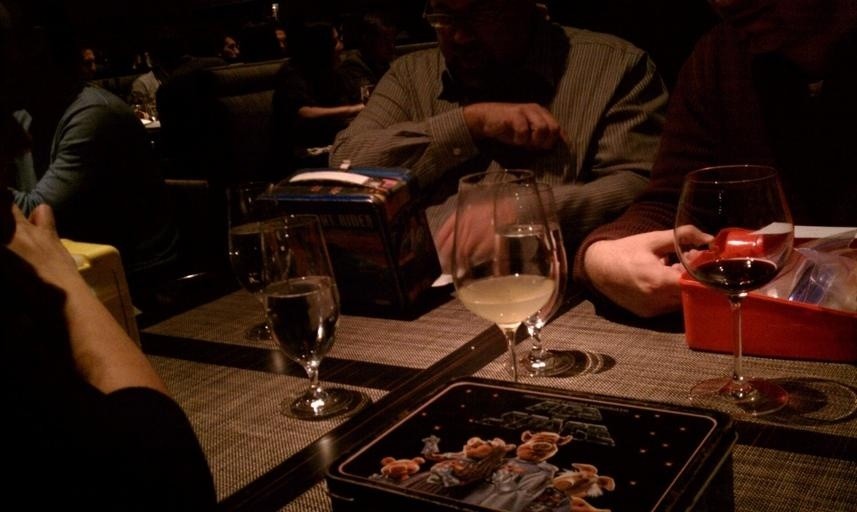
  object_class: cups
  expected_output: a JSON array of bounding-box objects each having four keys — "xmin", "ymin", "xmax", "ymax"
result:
[{"xmin": 146, "ymin": 103, "xmax": 159, "ymax": 121}]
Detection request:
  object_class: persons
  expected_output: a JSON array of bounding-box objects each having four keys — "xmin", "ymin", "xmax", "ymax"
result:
[
  {"xmin": 0, "ymin": 0, "xmax": 440, "ymax": 327},
  {"xmin": 1, "ymin": 184, "xmax": 218, "ymax": 512},
  {"xmin": 329, "ymin": 0, "xmax": 673, "ymax": 273},
  {"xmin": 572, "ymin": 1, "xmax": 856, "ymax": 319}
]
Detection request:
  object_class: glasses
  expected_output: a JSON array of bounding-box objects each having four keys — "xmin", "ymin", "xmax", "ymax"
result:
[{"xmin": 419, "ymin": 2, "xmax": 507, "ymax": 30}]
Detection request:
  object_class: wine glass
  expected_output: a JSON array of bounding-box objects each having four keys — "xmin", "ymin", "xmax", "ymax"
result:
[
  {"xmin": 673, "ymin": 166, "xmax": 794, "ymax": 420},
  {"xmin": 451, "ymin": 169, "xmax": 576, "ymax": 383},
  {"xmin": 224, "ymin": 215, "xmax": 372, "ymax": 423}
]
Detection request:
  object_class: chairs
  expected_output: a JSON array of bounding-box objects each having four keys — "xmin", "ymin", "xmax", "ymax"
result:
[{"xmin": 192, "ymin": 56, "xmax": 293, "ymax": 132}]
